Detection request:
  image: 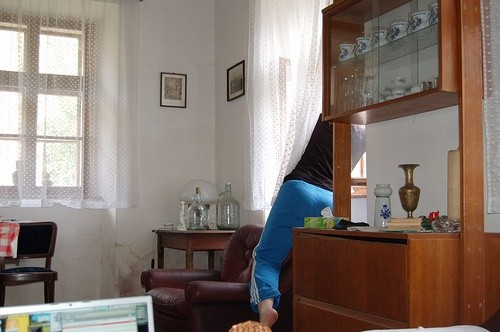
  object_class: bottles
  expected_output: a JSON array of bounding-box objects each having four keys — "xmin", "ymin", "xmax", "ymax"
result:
[
  {"xmin": 216, "ymin": 182, "xmax": 240, "ymax": 230},
  {"xmin": 186, "ymin": 186, "xmax": 208, "ymax": 230}
]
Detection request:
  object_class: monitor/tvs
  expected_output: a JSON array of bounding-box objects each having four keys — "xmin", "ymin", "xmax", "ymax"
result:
[{"xmin": 0, "ymin": 296, "xmax": 155, "ymax": 332}]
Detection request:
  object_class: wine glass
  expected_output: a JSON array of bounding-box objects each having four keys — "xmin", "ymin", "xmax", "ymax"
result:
[{"xmin": 334, "ymin": 74, "xmax": 375, "ymax": 112}]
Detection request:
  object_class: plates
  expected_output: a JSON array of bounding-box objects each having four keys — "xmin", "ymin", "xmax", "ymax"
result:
[{"xmin": 354, "ymin": 227, "xmax": 388, "ymax": 232}]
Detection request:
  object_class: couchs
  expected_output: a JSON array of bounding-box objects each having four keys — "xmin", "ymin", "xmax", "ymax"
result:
[{"xmin": 140, "ymin": 224, "xmax": 293, "ymax": 332}]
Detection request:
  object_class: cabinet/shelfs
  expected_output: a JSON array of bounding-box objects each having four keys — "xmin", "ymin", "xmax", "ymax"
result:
[{"xmin": 292, "ymin": 1, "xmax": 482, "ymax": 332}]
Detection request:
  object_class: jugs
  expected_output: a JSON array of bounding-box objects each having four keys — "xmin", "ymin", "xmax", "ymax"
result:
[
  {"xmin": 387, "ymin": 11, "xmax": 430, "ymax": 42},
  {"xmin": 339, "ymin": 31, "xmax": 386, "ymax": 63},
  {"xmin": 429, "ymin": 1, "xmax": 438, "ymax": 25}
]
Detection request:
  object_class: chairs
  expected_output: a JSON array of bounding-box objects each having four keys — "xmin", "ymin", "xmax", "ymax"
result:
[{"xmin": 0, "ymin": 222, "xmax": 59, "ymax": 309}]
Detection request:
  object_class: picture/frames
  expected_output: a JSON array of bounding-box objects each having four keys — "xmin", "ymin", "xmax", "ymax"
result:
[
  {"xmin": 161, "ymin": 72, "xmax": 187, "ymax": 108},
  {"xmin": 226, "ymin": 60, "xmax": 246, "ymax": 101}
]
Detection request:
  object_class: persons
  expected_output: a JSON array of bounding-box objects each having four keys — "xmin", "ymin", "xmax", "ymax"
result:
[{"xmin": 249, "ymin": 113, "xmax": 367, "ymax": 328}]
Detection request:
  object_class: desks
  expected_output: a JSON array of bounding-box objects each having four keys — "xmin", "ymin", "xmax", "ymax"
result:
[{"xmin": 152, "ymin": 230, "xmax": 235, "ymax": 270}]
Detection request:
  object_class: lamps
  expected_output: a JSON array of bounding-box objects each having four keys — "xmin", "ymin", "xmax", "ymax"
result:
[{"xmin": 178, "ymin": 178, "xmax": 220, "ymax": 209}]
